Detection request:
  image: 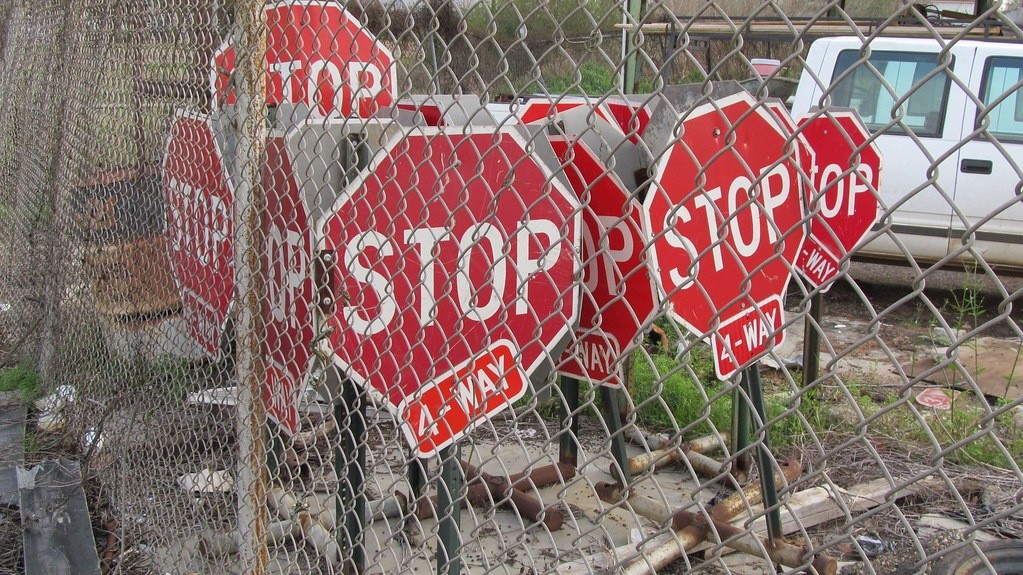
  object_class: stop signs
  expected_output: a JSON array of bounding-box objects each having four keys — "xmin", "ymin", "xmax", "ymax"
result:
[{"xmin": 161, "ymin": 1, "xmax": 886, "ymax": 458}]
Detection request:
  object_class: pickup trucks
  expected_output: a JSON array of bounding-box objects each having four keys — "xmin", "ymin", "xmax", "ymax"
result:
[{"xmin": 789, "ymin": 35, "xmax": 1023, "ymax": 278}]
